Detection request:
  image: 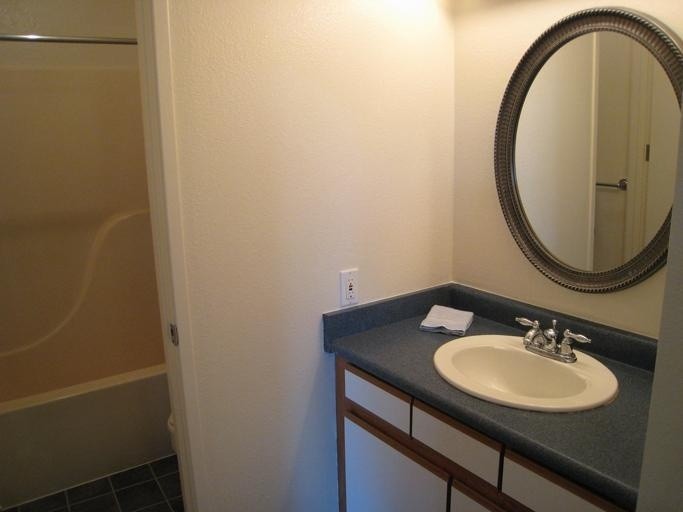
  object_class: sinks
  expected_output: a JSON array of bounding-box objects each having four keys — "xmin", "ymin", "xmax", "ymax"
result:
[{"xmin": 433, "ymin": 335, "xmax": 618, "ymax": 413}]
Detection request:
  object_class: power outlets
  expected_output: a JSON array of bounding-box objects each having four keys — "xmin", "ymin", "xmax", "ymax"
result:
[{"xmin": 339, "ymin": 268, "xmax": 359, "ymax": 308}]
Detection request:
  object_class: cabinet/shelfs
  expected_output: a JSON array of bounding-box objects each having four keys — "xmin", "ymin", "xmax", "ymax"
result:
[{"xmin": 336, "ymin": 363, "xmax": 618, "ymax": 511}]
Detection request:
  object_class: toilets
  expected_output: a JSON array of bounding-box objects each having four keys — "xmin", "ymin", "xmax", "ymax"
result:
[{"xmin": 167, "ymin": 413, "xmax": 177, "ymax": 453}]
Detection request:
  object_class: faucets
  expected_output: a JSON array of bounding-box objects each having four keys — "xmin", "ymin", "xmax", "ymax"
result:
[{"xmin": 542, "ymin": 319, "xmax": 560, "ymax": 360}]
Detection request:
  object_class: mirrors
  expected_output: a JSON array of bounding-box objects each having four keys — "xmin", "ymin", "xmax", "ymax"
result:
[{"xmin": 492, "ymin": 6, "xmax": 683, "ymax": 296}]
